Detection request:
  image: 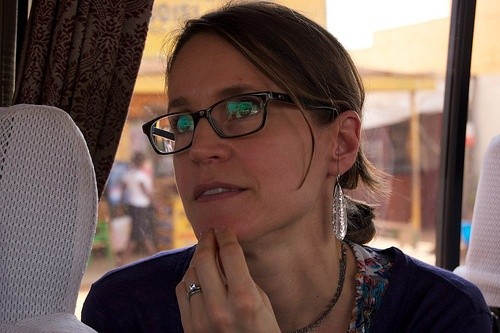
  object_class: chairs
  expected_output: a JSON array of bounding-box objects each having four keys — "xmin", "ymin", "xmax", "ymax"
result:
[
  {"xmin": 0, "ymin": 103, "xmax": 99, "ymax": 333},
  {"xmin": 86, "ymin": 219, "xmax": 113, "ymax": 264}
]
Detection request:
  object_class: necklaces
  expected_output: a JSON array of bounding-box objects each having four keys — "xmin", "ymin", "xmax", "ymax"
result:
[{"xmin": 286, "ymin": 242, "xmax": 348, "ymax": 333}]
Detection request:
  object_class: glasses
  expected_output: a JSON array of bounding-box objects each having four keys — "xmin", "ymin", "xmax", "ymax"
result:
[{"xmin": 142, "ymin": 91, "xmax": 348, "ymax": 155}]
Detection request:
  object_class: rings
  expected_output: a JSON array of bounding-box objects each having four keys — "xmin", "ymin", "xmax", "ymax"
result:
[{"xmin": 187, "ymin": 282, "xmax": 202, "ymax": 302}]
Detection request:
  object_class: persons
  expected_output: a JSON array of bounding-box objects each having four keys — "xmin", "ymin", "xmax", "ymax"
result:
[
  {"xmin": 119, "ymin": 151, "xmax": 157, "ymax": 257},
  {"xmin": 80, "ymin": 0, "xmax": 495, "ymax": 333}
]
